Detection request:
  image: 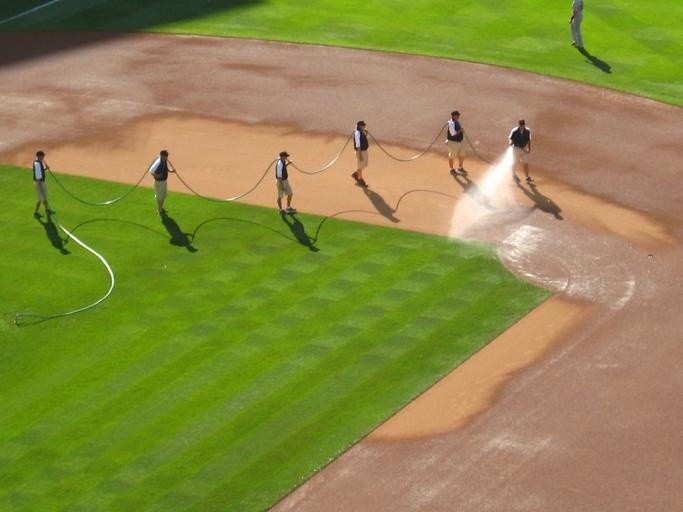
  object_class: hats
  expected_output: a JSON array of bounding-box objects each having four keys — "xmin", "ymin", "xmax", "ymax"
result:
[
  {"xmin": 452, "ymin": 111, "xmax": 460, "ymax": 117},
  {"xmin": 358, "ymin": 120, "xmax": 367, "ymax": 126},
  {"xmin": 281, "ymin": 152, "xmax": 290, "ymax": 158}
]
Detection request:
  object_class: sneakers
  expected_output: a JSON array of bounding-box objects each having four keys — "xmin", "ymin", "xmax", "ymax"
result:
[
  {"xmin": 279, "ymin": 207, "xmax": 296, "ymax": 213},
  {"xmin": 457, "ymin": 167, "xmax": 467, "ymax": 174},
  {"xmin": 352, "ymin": 171, "xmax": 368, "ymax": 188},
  {"xmin": 449, "ymin": 168, "xmax": 458, "ymax": 174}
]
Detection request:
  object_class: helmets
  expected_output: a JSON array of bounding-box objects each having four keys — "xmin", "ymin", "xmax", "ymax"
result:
[{"xmin": 36, "ymin": 150, "xmax": 45, "ymax": 155}]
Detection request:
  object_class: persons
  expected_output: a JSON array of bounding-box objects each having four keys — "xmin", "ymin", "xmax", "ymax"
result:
[
  {"xmin": 350, "ymin": 120, "xmax": 369, "ymax": 188},
  {"xmin": 149, "ymin": 149, "xmax": 175, "ymax": 215},
  {"xmin": 568, "ymin": 1, "xmax": 584, "ymax": 47},
  {"xmin": 442, "ymin": 111, "xmax": 468, "ymax": 175},
  {"xmin": 508, "ymin": 119, "xmax": 532, "ymax": 183},
  {"xmin": 32, "ymin": 150, "xmax": 55, "ymax": 217},
  {"xmin": 275, "ymin": 152, "xmax": 296, "ymax": 215}
]
